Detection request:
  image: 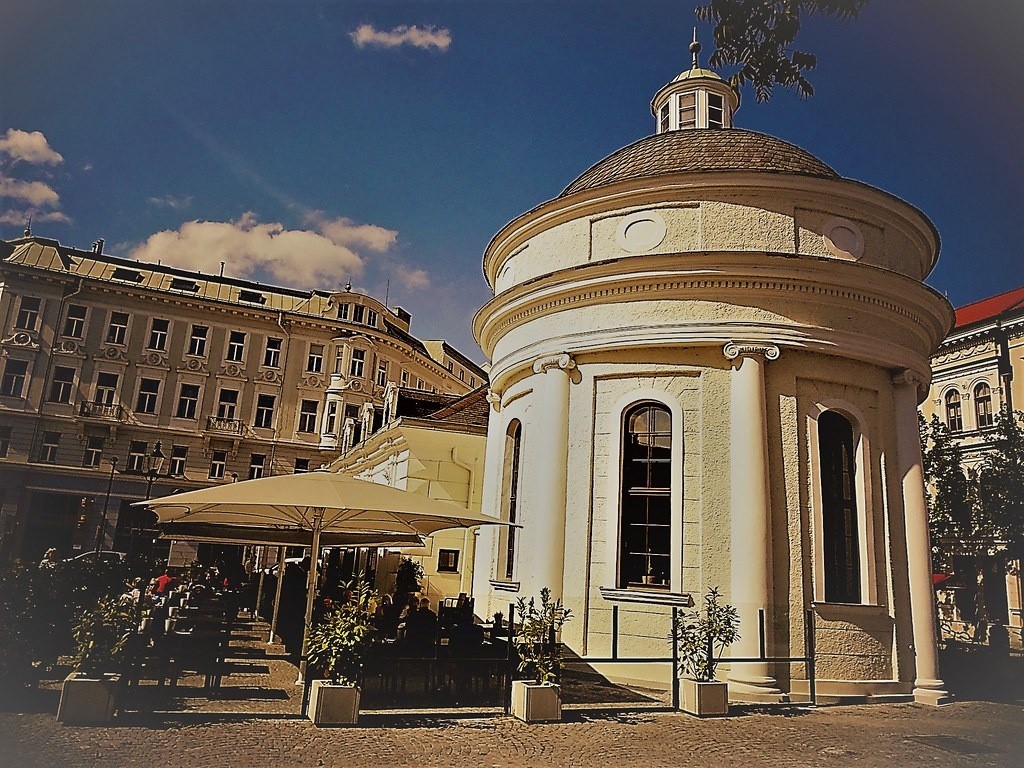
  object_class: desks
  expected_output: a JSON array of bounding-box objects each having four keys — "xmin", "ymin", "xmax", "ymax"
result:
[
  {"xmin": 129, "ymin": 573, "xmax": 244, "ymax": 698},
  {"xmin": 354, "ymin": 613, "xmax": 565, "ymax": 697}
]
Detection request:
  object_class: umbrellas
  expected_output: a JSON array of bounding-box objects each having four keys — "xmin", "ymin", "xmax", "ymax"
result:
[{"xmin": 129, "ymin": 471, "xmax": 523, "ymax": 685}]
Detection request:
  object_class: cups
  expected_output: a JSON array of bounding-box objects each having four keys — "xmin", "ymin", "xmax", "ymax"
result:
[{"xmin": 136, "ymin": 590, "xmax": 188, "ymax": 634}]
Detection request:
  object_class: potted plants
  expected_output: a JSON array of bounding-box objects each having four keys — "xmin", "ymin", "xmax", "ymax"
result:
[
  {"xmin": 512, "ymin": 587, "xmax": 575, "ymax": 721},
  {"xmin": 57, "ymin": 594, "xmax": 145, "ymax": 728},
  {"xmin": 303, "ymin": 570, "xmax": 381, "ymax": 726},
  {"xmin": 669, "ymin": 585, "xmax": 741, "ymax": 717}
]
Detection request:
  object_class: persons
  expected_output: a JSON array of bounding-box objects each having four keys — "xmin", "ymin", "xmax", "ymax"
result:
[
  {"xmin": 120, "ymin": 566, "xmax": 181, "ymax": 611},
  {"xmin": 400, "ymin": 597, "xmax": 485, "ymax": 651},
  {"xmin": 337, "ymin": 590, "xmax": 392, "ymax": 618}
]
